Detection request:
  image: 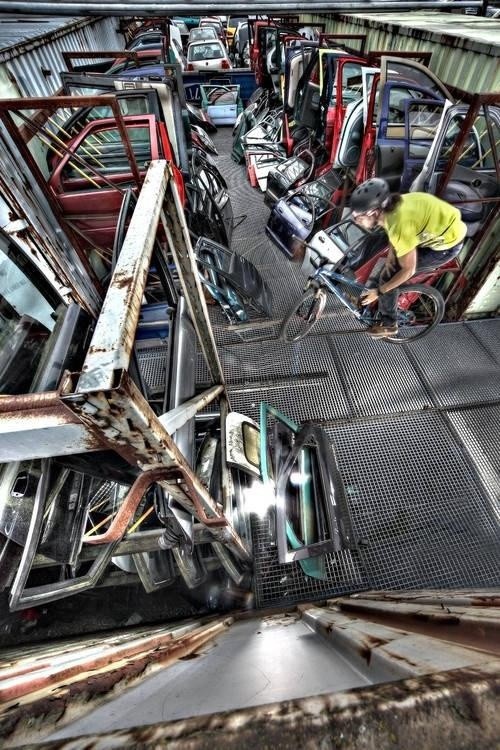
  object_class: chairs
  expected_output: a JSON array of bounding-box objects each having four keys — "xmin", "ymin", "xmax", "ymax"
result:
[
  {"xmin": 195, "ymin": 53, "xmax": 204, "ymax": 60},
  {"xmin": 214, "ymin": 50, "xmax": 221, "ymax": 57}
]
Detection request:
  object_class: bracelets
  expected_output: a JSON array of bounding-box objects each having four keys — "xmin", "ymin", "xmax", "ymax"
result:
[{"xmin": 376, "ymin": 286, "xmax": 383, "ymax": 298}]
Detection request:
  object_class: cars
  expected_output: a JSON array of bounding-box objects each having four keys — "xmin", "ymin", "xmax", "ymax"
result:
[{"xmin": 172, "ymin": 15, "xmax": 252, "ymax": 72}]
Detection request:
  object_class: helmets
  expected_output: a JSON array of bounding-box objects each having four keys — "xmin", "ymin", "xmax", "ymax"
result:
[{"xmin": 350, "ymin": 178, "xmax": 391, "ymax": 213}]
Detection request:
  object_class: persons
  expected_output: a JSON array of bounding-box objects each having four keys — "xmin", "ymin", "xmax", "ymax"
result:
[{"xmin": 348, "ymin": 176, "xmax": 468, "ymax": 338}]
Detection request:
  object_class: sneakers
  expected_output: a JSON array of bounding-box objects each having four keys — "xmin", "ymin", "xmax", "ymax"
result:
[
  {"xmin": 344, "ymin": 294, "xmax": 375, "ymax": 317},
  {"xmin": 365, "ymin": 322, "xmax": 399, "ymax": 337}
]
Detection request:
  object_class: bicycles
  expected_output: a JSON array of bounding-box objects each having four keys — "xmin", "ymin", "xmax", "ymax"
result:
[{"xmin": 278, "ymin": 232, "xmax": 449, "ymax": 346}]
